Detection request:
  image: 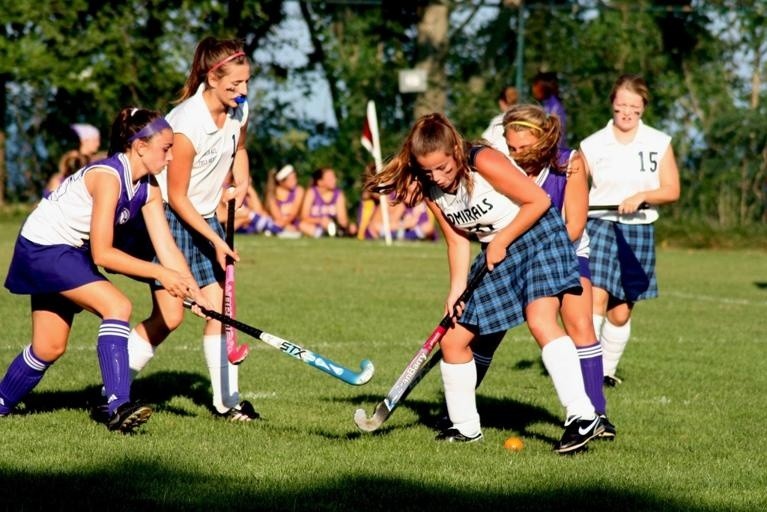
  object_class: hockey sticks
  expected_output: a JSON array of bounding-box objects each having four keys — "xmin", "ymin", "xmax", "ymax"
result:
[
  {"xmin": 182, "ymin": 298, "xmax": 374, "ymax": 385},
  {"xmin": 353, "ymin": 263, "xmax": 489, "ymax": 433},
  {"xmin": 223, "ymin": 186, "xmax": 250, "ymax": 366}
]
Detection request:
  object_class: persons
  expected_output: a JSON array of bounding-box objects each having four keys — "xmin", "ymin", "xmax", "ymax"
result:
[
  {"xmin": 358, "ymin": 111, "xmax": 604, "ymax": 453},
  {"xmin": 365, "ymin": 178, "xmax": 439, "ymax": 240},
  {"xmin": 45, "ymin": 123, "xmax": 100, "ymax": 192},
  {"xmin": 482, "ymin": 72, "xmax": 566, "ymax": 159},
  {"xmin": 89, "ymin": 37, "xmax": 263, "ymax": 426},
  {"xmin": 3, "ymin": 104, "xmax": 217, "ymax": 434},
  {"xmin": 469, "ymin": 104, "xmax": 619, "ymax": 444},
  {"xmin": 575, "ymin": 73, "xmax": 682, "ymax": 391},
  {"xmin": 224, "ymin": 163, "xmax": 355, "ymax": 237}
]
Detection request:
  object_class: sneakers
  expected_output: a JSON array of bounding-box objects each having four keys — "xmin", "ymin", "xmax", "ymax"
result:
[
  {"xmin": 551, "ymin": 414, "xmax": 606, "ymax": 453},
  {"xmin": 434, "ymin": 427, "xmax": 482, "ymax": 443},
  {"xmin": 604, "ymin": 375, "xmax": 622, "ymax": 386},
  {"xmin": 84, "ymin": 385, "xmax": 152, "ymax": 433},
  {"xmin": 213, "ymin": 400, "xmax": 268, "ymax": 422},
  {"xmin": 597, "ymin": 417, "xmax": 616, "ymax": 437}
]
